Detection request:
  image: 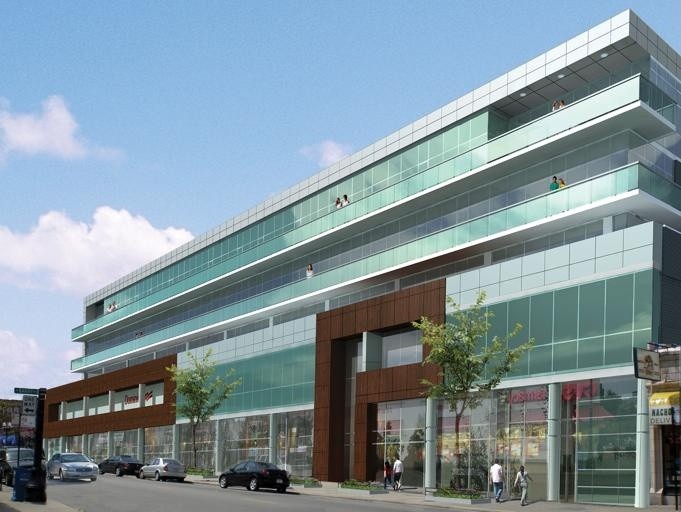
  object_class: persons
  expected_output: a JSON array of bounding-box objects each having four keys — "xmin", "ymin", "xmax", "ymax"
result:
[
  {"xmin": 383, "ymin": 460, "xmax": 396, "ymax": 491},
  {"xmin": 558, "ymin": 178, "xmax": 566, "ymax": 191},
  {"xmin": 139, "ymin": 331, "xmax": 145, "ymax": 337},
  {"xmin": 106, "ymin": 300, "xmax": 118, "ymax": 313},
  {"xmin": 306, "ymin": 263, "xmax": 314, "ymax": 280},
  {"xmin": 336, "ymin": 197, "xmax": 342, "ymax": 209},
  {"xmin": 341, "ymin": 195, "xmax": 350, "ymax": 206},
  {"xmin": 135, "ymin": 334, "xmax": 139, "ymax": 339},
  {"xmin": 552, "ymin": 100, "xmax": 559, "ymax": 112},
  {"xmin": 549, "ymin": 175, "xmax": 559, "ymax": 194},
  {"xmin": 391, "ymin": 456, "xmax": 404, "ymax": 490},
  {"xmin": 558, "ymin": 99, "xmax": 565, "ymax": 109},
  {"xmin": 513, "ymin": 465, "xmax": 533, "ymax": 506},
  {"xmin": 489, "ymin": 458, "xmax": 506, "ymax": 503}
]
